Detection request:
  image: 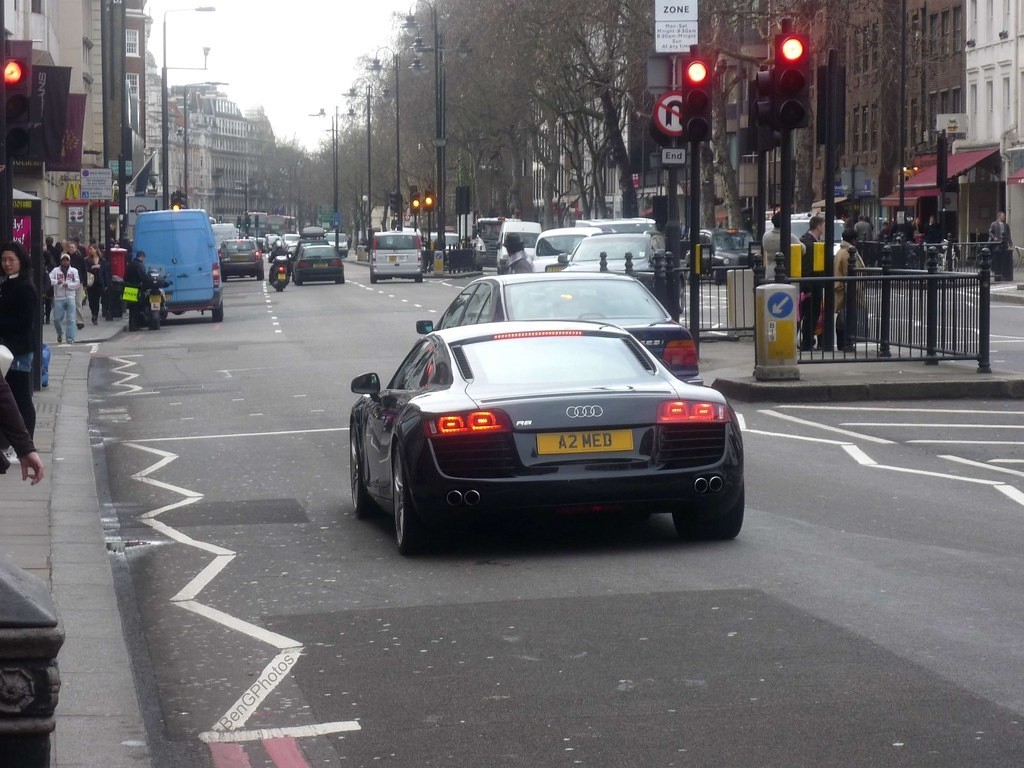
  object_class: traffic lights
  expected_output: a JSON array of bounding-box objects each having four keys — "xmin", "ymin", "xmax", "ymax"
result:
[
  {"xmin": 389, "ymin": 191, "xmax": 402, "ymax": 212},
  {"xmin": 773, "ymin": 33, "xmax": 811, "ymax": 129},
  {"xmin": 245, "ymin": 215, "xmax": 251, "ymax": 228},
  {"xmin": 424, "ymin": 186, "xmax": 434, "ymax": 212},
  {"xmin": 681, "ymin": 57, "xmax": 714, "ymax": 142},
  {"xmin": 0, "ymin": 56, "xmax": 32, "ymax": 153},
  {"xmin": 236, "ymin": 216, "xmax": 241, "ymax": 228},
  {"xmin": 409, "ymin": 186, "xmax": 421, "ymax": 213},
  {"xmin": 170, "ymin": 193, "xmax": 182, "ymax": 211}
]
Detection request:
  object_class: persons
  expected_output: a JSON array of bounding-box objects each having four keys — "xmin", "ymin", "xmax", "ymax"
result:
[
  {"xmin": 125, "ymin": 251, "xmax": 148, "ymax": 330},
  {"xmin": 42, "ymin": 233, "xmax": 131, "ymax": 329},
  {"xmin": 0, "ymin": 240, "xmax": 39, "ymax": 439},
  {"xmin": 0, "ymin": 369, "xmax": 45, "ymax": 486},
  {"xmin": 834, "ymin": 229, "xmax": 867, "ymax": 353},
  {"xmin": 761, "ymin": 211, "xmax": 806, "ymax": 284},
  {"xmin": 267, "ymin": 240, "xmax": 291, "ymax": 284},
  {"xmin": 799, "ymin": 216, "xmax": 825, "ymax": 350},
  {"xmin": 49, "ymin": 253, "xmax": 80, "ymax": 344},
  {"xmin": 987, "ymin": 211, "xmax": 1013, "ymax": 281},
  {"xmin": 845, "ymin": 214, "xmax": 941, "ymax": 272}
]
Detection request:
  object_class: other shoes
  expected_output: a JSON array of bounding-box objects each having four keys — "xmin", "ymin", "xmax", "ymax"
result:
[
  {"xmin": 77, "ymin": 324, "xmax": 84, "ymax": 330},
  {"xmin": 817, "ymin": 345, "xmax": 834, "ymax": 351},
  {"xmin": 57, "ymin": 336, "xmax": 62, "ymax": 343},
  {"xmin": 92, "ymin": 316, "xmax": 98, "ymax": 325},
  {"xmin": 1, "ymin": 444, "xmax": 20, "ymax": 464},
  {"xmin": 46, "ymin": 320, "xmax": 49, "ymax": 324},
  {"xmin": 66, "ymin": 338, "xmax": 73, "ymax": 344},
  {"xmin": 838, "ymin": 345, "xmax": 856, "ymax": 352},
  {"xmin": 800, "ymin": 339, "xmax": 816, "ymax": 350}
]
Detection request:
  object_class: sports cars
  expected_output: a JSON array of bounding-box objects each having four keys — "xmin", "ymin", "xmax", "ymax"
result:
[{"xmin": 348, "ymin": 318, "xmax": 745, "ymax": 560}]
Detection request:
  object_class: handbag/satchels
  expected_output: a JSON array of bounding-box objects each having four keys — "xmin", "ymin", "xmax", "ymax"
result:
[
  {"xmin": 87, "ymin": 271, "xmax": 94, "ymax": 287},
  {"xmin": 987, "ymin": 237, "xmax": 992, "ymax": 245},
  {"xmin": 120, "ymin": 286, "xmax": 139, "ymax": 303}
]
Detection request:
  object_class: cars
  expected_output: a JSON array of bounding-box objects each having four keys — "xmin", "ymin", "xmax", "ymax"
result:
[
  {"xmin": 558, "ymin": 231, "xmax": 687, "ymax": 315},
  {"xmin": 290, "ymin": 244, "xmax": 345, "ymax": 286},
  {"xmin": 211, "ymin": 223, "xmax": 349, "ymax": 258},
  {"xmin": 416, "ymin": 270, "xmax": 704, "ymax": 387}
]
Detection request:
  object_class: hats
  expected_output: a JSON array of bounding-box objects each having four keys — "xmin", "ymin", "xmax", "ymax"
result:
[
  {"xmin": 73, "ymin": 237, "xmax": 80, "ymax": 242},
  {"xmin": 60, "ymin": 254, "xmax": 70, "ymax": 260}
]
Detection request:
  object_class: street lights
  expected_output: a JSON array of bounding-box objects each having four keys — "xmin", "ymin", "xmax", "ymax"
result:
[
  {"xmin": 400, "ymin": 0, "xmax": 460, "ymax": 250},
  {"xmin": 365, "ymin": 46, "xmax": 427, "ymax": 231},
  {"xmin": 346, "ymin": 76, "xmax": 392, "ymax": 253},
  {"xmin": 184, "ymin": 81, "xmax": 230, "ymax": 193},
  {"xmin": 408, "ymin": 32, "xmax": 476, "ymax": 248},
  {"xmin": 315, "ymin": 104, "xmax": 358, "ymax": 254},
  {"xmin": 161, "ymin": 6, "xmax": 218, "ymax": 211}
]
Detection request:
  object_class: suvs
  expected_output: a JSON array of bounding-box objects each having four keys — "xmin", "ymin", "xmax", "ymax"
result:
[
  {"xmin": 217, "ymin": 238, "xmax": 267, "ymax": 281},
  {"xmin": 687, "ymin": 227, "xmax": 764, "ymax": 285}
]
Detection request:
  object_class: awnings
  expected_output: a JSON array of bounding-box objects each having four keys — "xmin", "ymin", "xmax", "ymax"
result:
[
  {"xmin": 880, "ymin": 192, "xmax": 920, "ymax": 206},
  {"xmin": 1008, "ymin": 167, "xmax": 1024, "ymax": 184},
  {"xmin": 894, "ymin": 149, "xmax": 999, "ymax": 196},
  {"xmin": 811, "ymin": 197, "xmax": 846, "ymax": 208}
]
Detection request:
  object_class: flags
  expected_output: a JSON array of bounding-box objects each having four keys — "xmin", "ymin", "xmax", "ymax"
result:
[
  {"xmin": 6, "ymin": 40, "xmax": 87, "ymax": 172},
  {"xmin": 137, "ymin": 158, "xmax": 152, "ymax": 192}
]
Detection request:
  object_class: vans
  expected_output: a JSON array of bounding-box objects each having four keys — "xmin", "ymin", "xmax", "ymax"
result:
[
  {"xmin": 764, "ymin": 212, "xmax": 846, "ymax": 259},
  {"xmin": 128, "ymin": 208, "xmax": 226, "ymax": 323},
  {"xmin": 359, "ymin": 217, "xmax": 661, "ymax": 275},
  {"xmin": 364, "ymin": 227, "xmax": 430, "ymax": 284}
]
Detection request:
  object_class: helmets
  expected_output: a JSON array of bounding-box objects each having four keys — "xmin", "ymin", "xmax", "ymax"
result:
[{"xmin": 275, "ymin": 241, "xmax": 282, "ymax": 248}]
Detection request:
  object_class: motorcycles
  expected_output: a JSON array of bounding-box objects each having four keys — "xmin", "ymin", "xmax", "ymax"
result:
[
  {"xmin": 143, "ymin": 266, "xmax": 173, "ymax": 331},
  {"xmin": 268, "ymin": 253, "xmax": 292, "ymax": 292}
]
